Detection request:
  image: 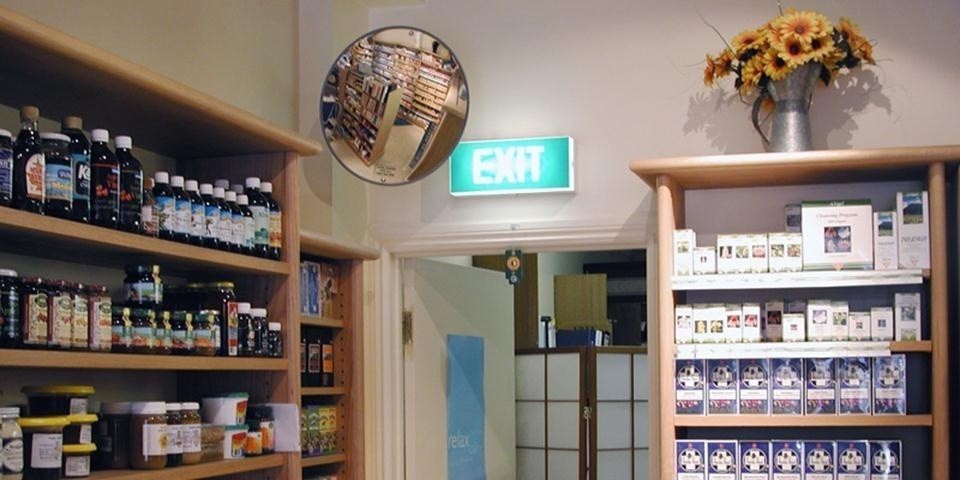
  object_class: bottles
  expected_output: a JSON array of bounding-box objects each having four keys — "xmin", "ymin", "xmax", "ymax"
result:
[
  {"xmin": 0, "ymin": 104, "xmax": 283, "ymax": 261},
  {"xmin": 99, "ymin": 399, "xmax": 204, "ymax": 470},
  {"xmin": 0, "ymin": 407, "xmax": 26, "ymax": 480},
  {"xmin": 242, "ymin": 406, "xmax": 275, "ymax": 456},
  {"xmin": 300, "ymin": 334, "xmax": 335, "ymax": 387}
]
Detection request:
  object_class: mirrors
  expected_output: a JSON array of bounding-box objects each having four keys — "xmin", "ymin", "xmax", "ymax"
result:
[{"xmin": 320, "ymin": 25, "xmax": 470, "ymax": 186}]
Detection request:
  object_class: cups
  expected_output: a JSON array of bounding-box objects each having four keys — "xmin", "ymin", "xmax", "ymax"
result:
[
  {"xmin": 201, "ymin": 391, "xmax": 249, "ymax": 459},
  {"xmin": 18, "ymin": 382, "xmax": 100, "ymax": 480}
]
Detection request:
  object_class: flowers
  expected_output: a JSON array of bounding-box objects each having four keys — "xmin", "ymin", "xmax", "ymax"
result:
[{"xmin": 704, "ymin": 1, "xmax": 875, "ymax": 107}]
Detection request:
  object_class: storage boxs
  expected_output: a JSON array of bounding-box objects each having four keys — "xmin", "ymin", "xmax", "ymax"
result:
[{"xmin": 801, "ymin": 199, "xmax": 873, "ymax": 271}]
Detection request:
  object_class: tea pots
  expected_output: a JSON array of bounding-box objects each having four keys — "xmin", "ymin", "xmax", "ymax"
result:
[{"xmin": 752, "ymin": 64, "xmax": 824, "ymax": 152}]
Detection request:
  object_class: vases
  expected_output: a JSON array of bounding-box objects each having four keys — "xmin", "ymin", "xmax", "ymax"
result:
[{"xmin": 765, "ymin": 62, "xmax": 817, "ymax": 151}]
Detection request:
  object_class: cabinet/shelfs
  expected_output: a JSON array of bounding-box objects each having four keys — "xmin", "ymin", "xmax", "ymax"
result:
[
  {"xmin": 299, "ymin": 229, "xmax": 378, "ymax": 479},
  {"xmin": 629, "ymin": 144, "xmax": 960, "ymax": 480},
  {"xmin": 0, "ymin": 8, "xmax": 322, "ymax": 479},
  {"xmin": 329, "ymin": 40, "xmax": 469, "ymax": 184}
]
